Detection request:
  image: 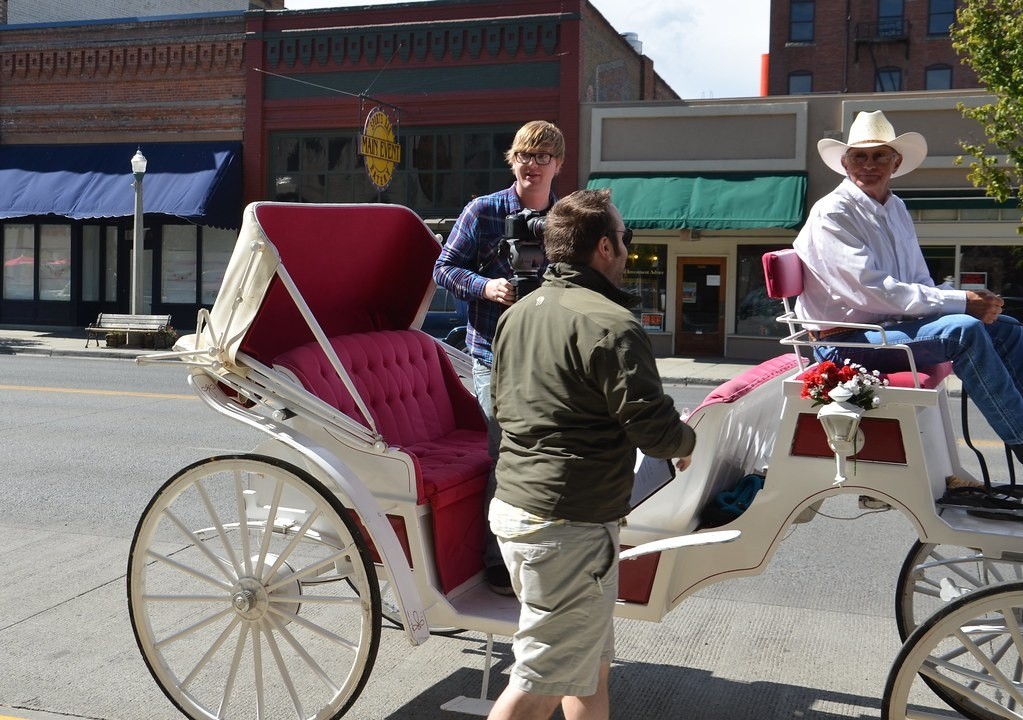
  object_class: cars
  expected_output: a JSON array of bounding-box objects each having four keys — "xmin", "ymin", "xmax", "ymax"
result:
[{"xmin": 123, "ymin": 201, "xmax": 1023, "ymax": 720}]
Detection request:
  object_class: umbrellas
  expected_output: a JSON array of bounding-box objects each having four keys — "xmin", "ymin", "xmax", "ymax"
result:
[{"xmin": 5, "ymin": 253, "xmax": 34, "ymax": 282}]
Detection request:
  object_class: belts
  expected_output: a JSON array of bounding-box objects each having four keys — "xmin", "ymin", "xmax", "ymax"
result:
[{"xmin": 809, "ymin": 326, "xmax": 858, "ymax": 342}]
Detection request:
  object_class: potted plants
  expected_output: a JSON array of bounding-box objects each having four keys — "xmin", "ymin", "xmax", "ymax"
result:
[
  {"xmin": 144, "ymin": 333, "xmax": 153, "ymax": 347},
  {"xmin": 105, "ymin": 332, "xmax": 126, "ymax": 347}
]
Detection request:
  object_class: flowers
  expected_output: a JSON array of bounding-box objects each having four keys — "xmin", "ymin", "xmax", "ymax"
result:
[
  {"xmin": 157, "ymin": 324, "xmax": 179, "ymax": 346},
  {"xmin": 798, "ymin": 358, "xmax": 889, "ymax": 411}
]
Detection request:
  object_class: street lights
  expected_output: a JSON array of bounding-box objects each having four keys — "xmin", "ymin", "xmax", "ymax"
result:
[{"xmin": 130, "ymin": 147, "xmax": 147, "ymax": 316}]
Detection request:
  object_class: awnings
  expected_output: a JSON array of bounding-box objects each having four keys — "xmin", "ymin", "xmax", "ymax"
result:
[
  {"xmin": 585, "ymin": 175, "xmax": 809, "ymax": 230},
  {"xmin": 1, "ymin": 139, "xmax": 242, "ymax": 223}
]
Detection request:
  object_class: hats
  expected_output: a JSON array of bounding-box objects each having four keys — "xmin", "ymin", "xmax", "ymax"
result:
[{"xmin": 817, "ymin": 109, "xmax": 928, "ymax": 180}]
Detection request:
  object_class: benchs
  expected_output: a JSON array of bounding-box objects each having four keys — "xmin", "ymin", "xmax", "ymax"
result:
[
  {"xmin": 762, "ymin": 245, "xmax": 956, "ymax": 507},
  {"xmin": 84, "ymin": 313, "xmax": 172, "ymax": 350},
  {"xmin": 278, "ymin": 328, "xmax": 499, "ymax": 598}
]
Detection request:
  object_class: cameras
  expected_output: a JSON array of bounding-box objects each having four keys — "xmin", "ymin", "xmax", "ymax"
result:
[{"xmin": 505, "ymin": 208, "xmax": 548, "ymax": 243}]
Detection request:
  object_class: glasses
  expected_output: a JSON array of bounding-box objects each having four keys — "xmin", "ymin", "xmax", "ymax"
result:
[
  {"xmin": 608, "ymin": 228, "xmax": 634, "ymax": 246},
  {"xmin": 514, "ymin": 151, "xmax": 559, "ymax": 165},
  {"xmin": 841, "ymin": 151, "xmax": 899, "ymax": 166}
]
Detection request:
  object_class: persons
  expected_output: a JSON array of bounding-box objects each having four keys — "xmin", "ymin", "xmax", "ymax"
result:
[
  {"xmin": 488, "ymin": 186, "xmax": 697, "ymax": 720},
  {"xmin": 792, "ymin": 110, "xmax": 1023, "ymax": 472},
  {"xmin": 433, "ymin": 120, "xmax": 567, "ymax": 594}
]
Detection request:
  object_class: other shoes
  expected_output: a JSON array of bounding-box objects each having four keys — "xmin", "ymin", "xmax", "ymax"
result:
[{"xmin": 485, "ymin": 563, "xmax": 516, "ymax": 596}]
